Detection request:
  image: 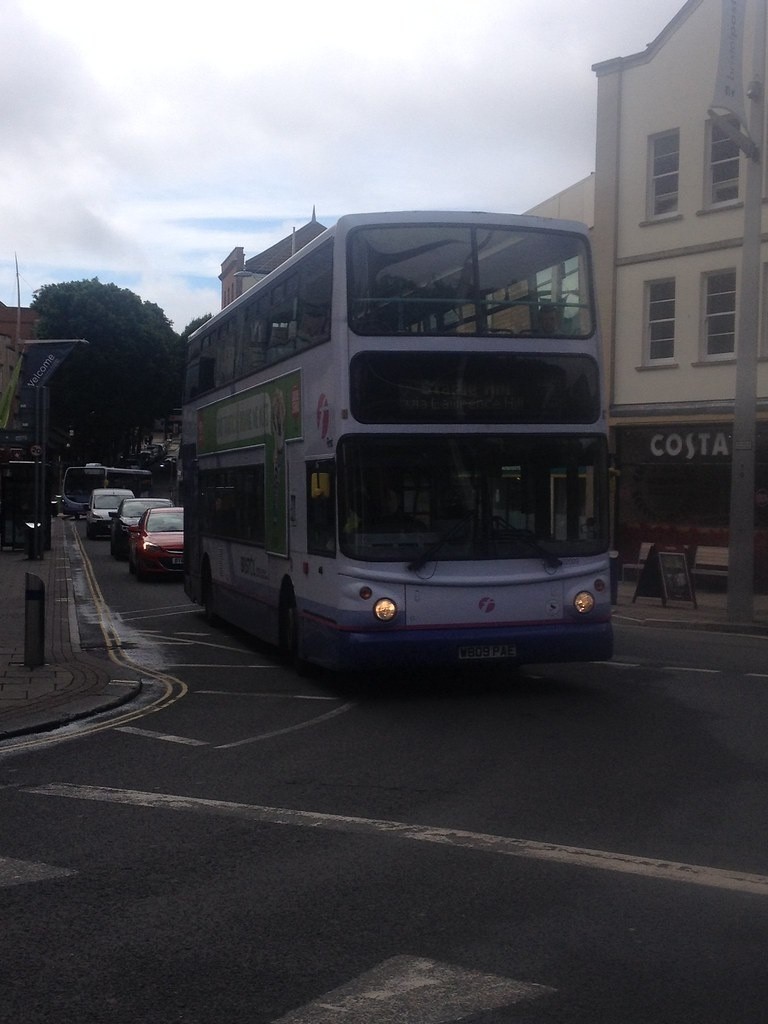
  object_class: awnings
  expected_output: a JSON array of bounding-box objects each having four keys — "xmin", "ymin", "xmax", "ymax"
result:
[{"xmin": 20, "ymin": 340, "xmax": 94, "ymax": 388}]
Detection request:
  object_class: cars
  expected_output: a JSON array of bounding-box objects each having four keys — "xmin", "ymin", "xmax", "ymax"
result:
[
  {"xmin": 83, "ymin": 487, "xmax": 135, "ymax": 539},
  {"xmin": 115, "ymin": 435, "xmax": 181, "ymax": 479},
  {"xmin": 128, "ymin": 507, "xmax": 214, "ymax": 583},
  {"xmin": 108, "ymin": 497, "xmax": 177, "ymax": 560}
]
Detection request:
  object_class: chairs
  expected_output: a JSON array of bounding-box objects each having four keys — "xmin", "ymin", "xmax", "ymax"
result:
[{"xmin": 482, "ymin": 328, "xmax": 542, "ymax": 336}]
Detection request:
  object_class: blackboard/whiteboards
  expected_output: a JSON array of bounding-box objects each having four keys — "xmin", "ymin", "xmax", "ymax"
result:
[{"xmin": 658, "ymin": 550, "xmax": 695, "ymax": 602}]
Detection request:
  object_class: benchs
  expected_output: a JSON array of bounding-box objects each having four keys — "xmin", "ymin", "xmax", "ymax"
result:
[{"xmin": 621, "ymin": 542, "xmax": 729, "ymax": 584}]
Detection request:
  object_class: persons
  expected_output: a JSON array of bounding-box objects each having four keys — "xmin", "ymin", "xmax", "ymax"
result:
[
  {"xmin": 539, "ymin": 304, "xmax": 566, "ymax": 337},
  {"xmin": 553, "ymin": 493, "xmax": 594, "ymax": 549}
]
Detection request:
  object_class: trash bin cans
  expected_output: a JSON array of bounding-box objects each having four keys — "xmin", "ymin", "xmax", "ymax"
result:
[
  {"xmin": 54, "ymin": 495, "xmax": 62, "ymax": 513},
  {"xmin": 608, "ymin": 550, "xmax": 621, "ymax": 606},
  {"xmin": 26, "ymin": 522, "xmax": 44, "ymax": 560},
  {"xmin": 51, "ymin": 501, "xmax": 58, "ymax": 518}
]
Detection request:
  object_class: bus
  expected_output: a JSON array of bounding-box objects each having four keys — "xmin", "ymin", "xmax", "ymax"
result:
[
  {"xmin": 177, "ymin": 207, "xmax": 623, "ymax": 687},
  {"xmin": 61, "ymin": 463, "xmax": 153, "ymax": 520}
]
